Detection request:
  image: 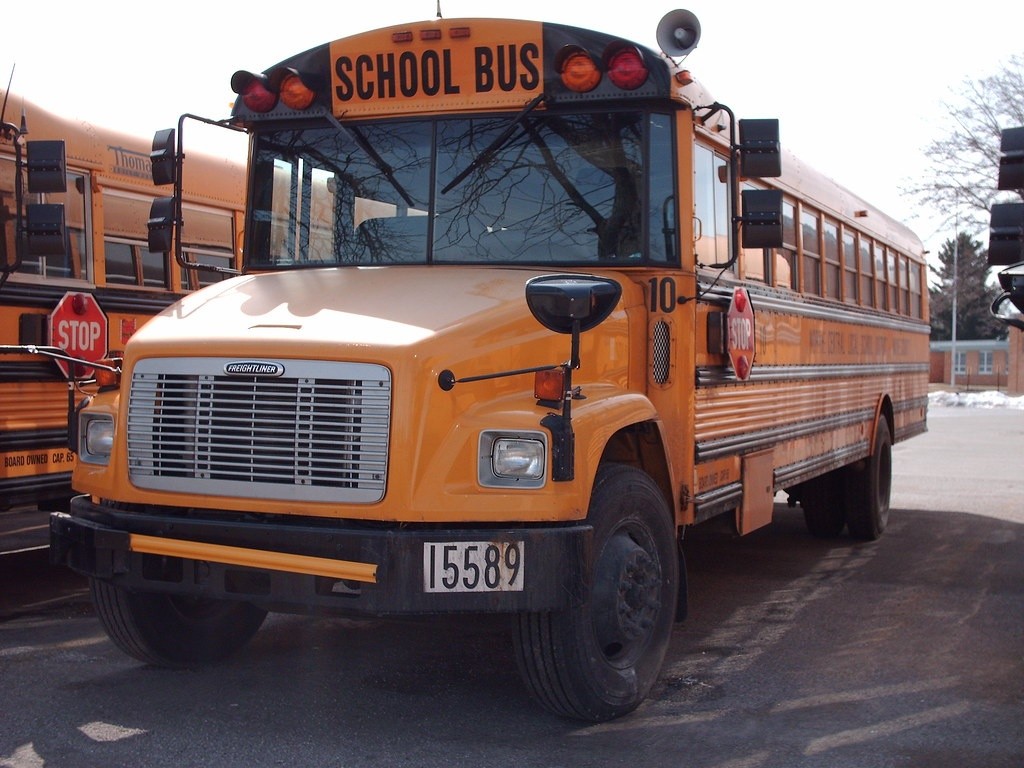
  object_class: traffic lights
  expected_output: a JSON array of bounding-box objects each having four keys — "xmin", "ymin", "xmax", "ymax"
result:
[{"xmin": 985, "ymin": 123, "xmax": 1024, "ymax": 269}]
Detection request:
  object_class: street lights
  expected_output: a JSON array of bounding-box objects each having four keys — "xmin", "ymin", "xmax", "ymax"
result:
[{"xmin": 933, "ymin": 182, "xmax": 961, "ymax": 385}]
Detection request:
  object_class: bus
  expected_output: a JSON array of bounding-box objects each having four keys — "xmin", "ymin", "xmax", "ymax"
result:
[
  {"xmin": 0, "ymin": 85, "xmax": 440, "ymax": 585},
  {"xmin": 2, "ymin": 0, "xmax": 936, "ymax": 723}
]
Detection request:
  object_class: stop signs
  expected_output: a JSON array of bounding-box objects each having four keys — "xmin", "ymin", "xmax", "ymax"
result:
[
  {"xmin": 50, "ymin": 292, "xmax": 109, "ymax": 379},
  {"xmin": 725, "ymin": 286, "xmax": 757, "ymax": 382}
]
